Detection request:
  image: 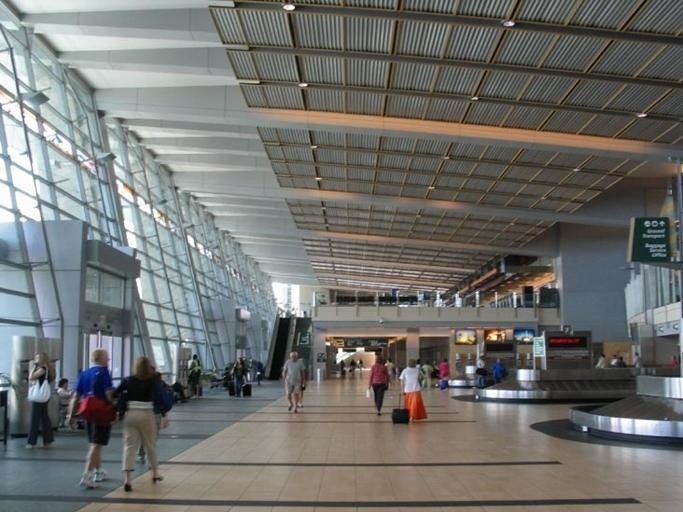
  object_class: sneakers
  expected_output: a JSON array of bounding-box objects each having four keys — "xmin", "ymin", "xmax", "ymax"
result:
[{"xmin": 80, "ymin": 469, "xmax": 107, "ymax": 487}]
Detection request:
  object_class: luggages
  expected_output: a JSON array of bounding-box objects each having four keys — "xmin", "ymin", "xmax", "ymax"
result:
[
  {"xmin": 392, "ymin": 393, "xmax": 410, "ymax": 424},
  {"xmin": 228, "ymin": 373, "xmax": 236, "ymax": 396},
  {"xmin": 242, "ymin": 371, "xmax": 252, "ymax": 396}
]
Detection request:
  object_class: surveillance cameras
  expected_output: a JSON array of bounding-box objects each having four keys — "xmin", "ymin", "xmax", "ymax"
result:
[{"xmin": 378, "ymin": 318, "xmax": 384, "ymax": 324}]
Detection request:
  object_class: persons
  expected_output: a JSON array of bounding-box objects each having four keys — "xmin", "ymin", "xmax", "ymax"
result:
[
  {"xmin": 58, "ymin": 349, "xmax": 187, "ymax": 491},
  {"xmin": 232, "ymin": 357, "xmax": 249, "ymax": 397},
  {"xmin": 257, "ymin": 361, "xmax": 264, "ymax": 385},
  {"xmin": 294, "ymin": 352, "xmax": 306, "ymax": 408},
  {"xmin": 368, "ymin": 355, "xmax": 507, "ymax": 424},
  {"xmin": 24, "ymin": 352, "xmax": 55, "ymax": 448},
  {"xmin": 339, "ymin": 358, "xmax": 363, "ymax": 377},
  {"xmin": 596, "ymin": 351, "xmax": 642, "ymax": 369},
  {"xmin": 671, "ymin": 355, "xmax": 679, "ymax": 368},
  {"xmin": 281, "ymin": 352, "xmax": 305, "ymax": 412},
  {"xmin": 189, "ymin": 353, "xmax": 202, "ymax": 399}
]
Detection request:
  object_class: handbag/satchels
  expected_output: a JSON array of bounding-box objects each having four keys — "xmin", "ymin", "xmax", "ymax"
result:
[
  {"xmin": 476, "ymin": 368, "xmax": 487, "ymax": 375},
  {"xmin": 27, "ymin": 380, "xmax": 52, "ymax": 403},
  {"xmin": 77, "ymin": 393, "xmax": 117, "ymax": 421},
  {"xmin": 365, "ymin": 388, "xmax": 371, "ymax": 398}
]
